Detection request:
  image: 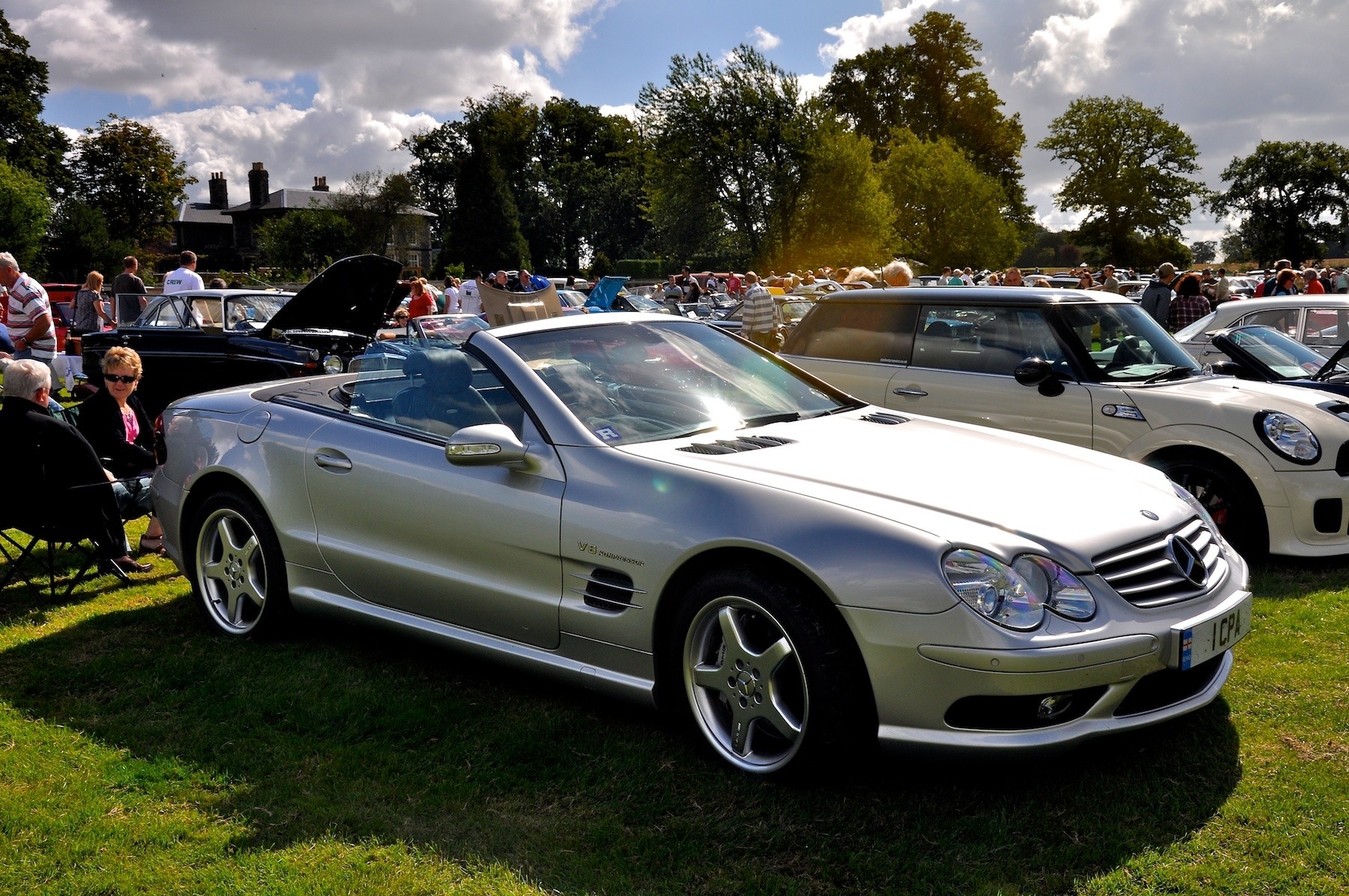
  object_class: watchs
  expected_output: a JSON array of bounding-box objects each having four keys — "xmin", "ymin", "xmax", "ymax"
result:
[{"xmin": 22, "ymin": 336, "xmax": 28, "ymax": 344}]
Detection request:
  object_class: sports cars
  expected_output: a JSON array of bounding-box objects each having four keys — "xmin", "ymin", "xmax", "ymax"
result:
[
  {"xmin": 144, "ymin": 309, "xmax": 1254, "ymax": 789},
  {"xmin": 1203, "ymin": 323, "xmax": 1349, "ymax": 398}
]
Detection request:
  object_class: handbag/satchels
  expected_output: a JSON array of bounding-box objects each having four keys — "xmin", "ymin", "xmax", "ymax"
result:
[{"xmin": 65, "ymin": 327, "xmax": 76, "ymax": 337}]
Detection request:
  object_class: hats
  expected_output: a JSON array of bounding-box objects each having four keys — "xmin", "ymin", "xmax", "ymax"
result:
[{"xmin": 1159, "ymin": 262, "xmax": 1179, "ymax": 278}]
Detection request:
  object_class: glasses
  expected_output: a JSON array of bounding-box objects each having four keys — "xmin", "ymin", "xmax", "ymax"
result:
[
  {"xmin": 1082, "ymin": 277, "xmax": 1088, "ymax": 279},
  {"xmin": 681, "ymin": 271, "xmax": 687, "ymax": 274},
  {"xmin": 1105, "ymin": 270, "xmax": 1108, "ymax": 272},
  {"xmin": 398, "ymin": 316, "xmax": 408, "ymax": 319},
  {"xmin": 104, "ymin": 369, "xmax": 138, "ymax": 384},
  {"xmin": 835, "ymin": 276, "xmax": 840, "ymax": 279},
  {"xmin": 525, "ymin": 275, "xmax": 530, "ymax": 278},
  {"xmin": 499, "ymin": 276, "xmax": 508, "ymax": 279},
  {"xmin": 1282, "ymin": 266, "xmax": 1293, "ymax": 270},
  {"xmin": 1288, "ymin": 278, "xmax": 1295, "ymax": 283}
]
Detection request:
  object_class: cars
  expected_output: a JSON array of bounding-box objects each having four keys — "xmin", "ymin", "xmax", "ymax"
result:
[
  {"xmin": 1088, "ymin": 290, "xmax": 1349, "ymax": 369},
  {"xmin": 760, "ymin": 282, "xmax": 1349, "ymax": 570},
  {"xmin": 78, "ymin": 252, "xmax": 404, "ymax": 424},
  {"xmin": 0, "ymin": 281, "xmax": 118, "ymax": 356},
  {"xmin": 348, "ymin": 267, "xmax": 1349, "ymax": 365}
]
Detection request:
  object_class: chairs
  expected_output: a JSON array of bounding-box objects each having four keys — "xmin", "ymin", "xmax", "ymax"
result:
[
  {"xmin": 392, "ymin": 348, "xmax": 504, "ymax": 446},
  {"xmin": 0, "ymin": 353, "xmax": 154, "ymax": 606},
  {"xmin": 1211, "ymin": 359, "xmax": 1245, "ymax": 378}
]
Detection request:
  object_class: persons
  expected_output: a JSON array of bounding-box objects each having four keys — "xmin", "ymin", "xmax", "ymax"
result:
[
  {"xmin": 741, "ymin": 271, "xmax": 777, "ymax": 352},
  {"xmin": 1255, "ymin": 259, "xmax": 1349, "ymax": 333},
  {"xmin": 1141, "ymin": 262, "xmax": 1229, "ymax": 334},
  {"xmin": 589, "ymin": 276, "xmax": 600, "ymax": 289},
  {"xmin": 1070, "ymin": 264, "xmax": 1137, "ymax": 351},
  {"xmin": 443, "ymin": 269, "xmax": 538, "ymax": 325},
  {"xmin": 1232, "ymin": 270, "xmax": 1238, "ymax": 277},
  {"xmin": 704, "ymin": 268, "xmax": 777, "ymax": 302},
  {"xmin": 564, "ymin": 276, "xmax": 576, "ymax": 290},
  {"xmin": 936, "ymin": 266, "xmax": 1052, "ymax": 320},
  {"xmin": 783, "ymin": 261, "xmax": 914, "ymax": 294},
  {"xmin": 0, "ymin": 249, "xmax": 243, "ymax": 566},
  {"xmin": 652, "ymin": 266, "xmax": 702, "ymax": 315},
  {"xmin": 379, "ymin": 276, "xmax": 444, "ymax": 340}
]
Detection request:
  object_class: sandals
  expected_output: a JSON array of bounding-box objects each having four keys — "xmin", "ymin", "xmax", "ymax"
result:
[{"xmin": 138, "ymin": 533, "xmax": 165, "ymax": 554}]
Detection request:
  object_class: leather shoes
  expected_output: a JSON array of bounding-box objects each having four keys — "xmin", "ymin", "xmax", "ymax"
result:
[{"xmin": 98, "ymin": 556, "xmax": 155, "ymax": 574}]
